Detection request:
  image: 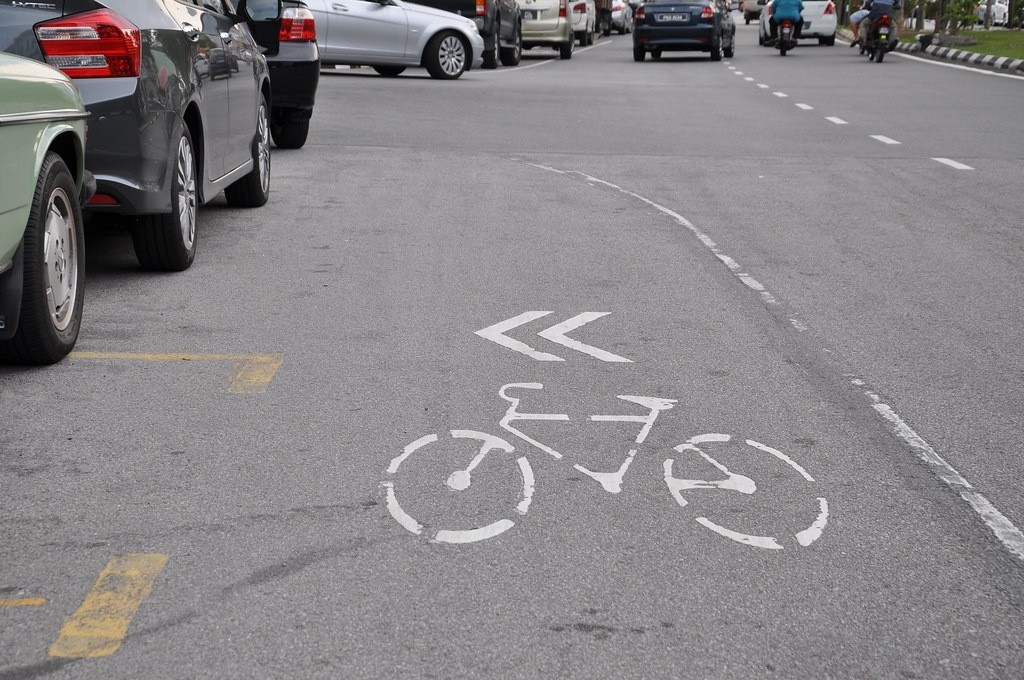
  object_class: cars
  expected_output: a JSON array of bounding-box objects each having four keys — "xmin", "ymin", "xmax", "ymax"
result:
[
  {"xmin": 978, "ymin": 0, "xmax": 996, "ymax": 26},
  {"xmin": 727, "ymin": 0, "xmax": 741, "ymax": 11},
  {"xmin": 758, "ymin": 0, "xmax": 837, "ymax": 48},
  {"xmin": 568, "ymin": 1, "xmax": 596, "ymax": 46},
  {"xmin": 301, "ymin": 0, "xmax": 485, "ymax": 81},
  {"xmin": 992, "ymin": 0, "xmax": 1010, "ymax": 26},
  {"xmin": 611, "ymin": 0, "xmax": 633, "ymax": 34},
  {"xmin": 231, "ymin": 0, "xmax": 319, "ymax": 150},
  {"xmin": 594, "ymin": 0, "xmax": 612, "ymax": 36},
  {"xmin": 631, "ymin": 0, "xmax": 735, "ymax": 63},
  {"xmin": 0, "ymin": 51, "xmax": 97, "ymax": 365},
  {"xmin": 515, "ymin": 0, "xmax": 573, "ymax": 59},
  {"xmin": 406, "ymin": 0, "xmax": 523, "ymax": 69},
  {"xmin": 0, "ymin": 0, "xmax": 273, "ymax": 270},
  {"xmin": 739, "ymin": 0, "xmax": 771, "ymax": 24}
]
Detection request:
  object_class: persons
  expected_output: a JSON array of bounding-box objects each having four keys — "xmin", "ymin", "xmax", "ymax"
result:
[
  {"xmin": 769, "ymin": 0, "xmax": 804, "ymax": 40},
  {"xmin": 848, "ymin": 1, "xmax": 903, "ymax": 49}
]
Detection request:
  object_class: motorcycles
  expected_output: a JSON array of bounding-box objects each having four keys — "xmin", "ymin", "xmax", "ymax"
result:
[
  {"xmin": 855, "ymin": 5, "xmax": 872, "ymax": 56},
  {"xmin": 862, "ymin": 5, "xmax": 896, "ymax": 62},
  {"xmin": 777, "ymin": 17, "xmax": 794, "ymax": 55}
]
